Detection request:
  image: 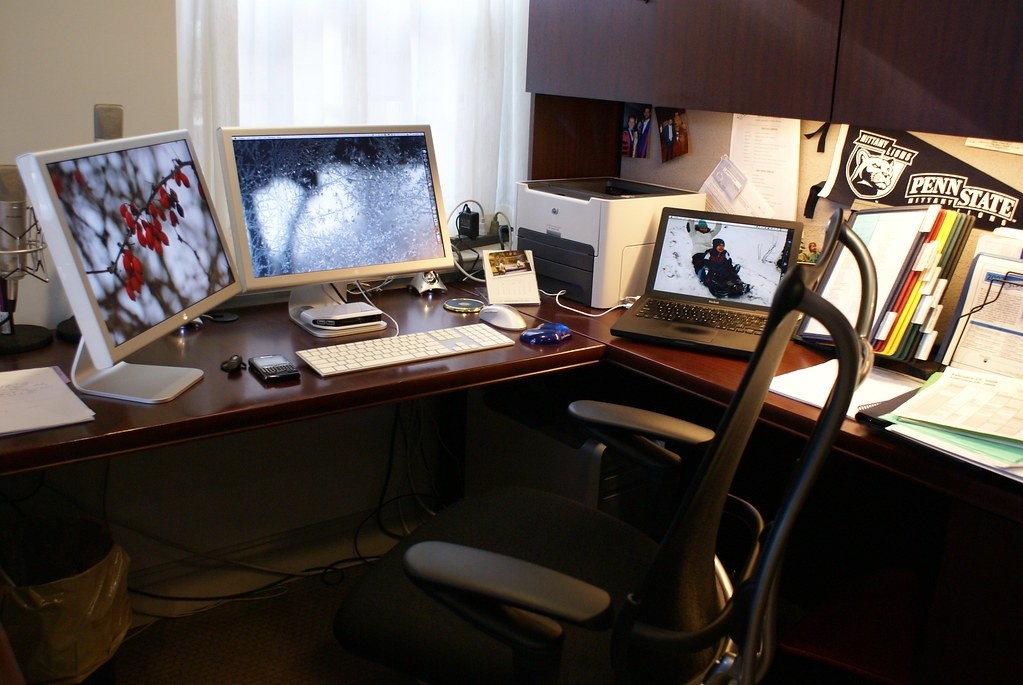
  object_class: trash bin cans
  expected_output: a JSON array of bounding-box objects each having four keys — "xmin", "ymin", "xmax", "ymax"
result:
[{"xmin": 0, "ymin": 513, "xmax": 131, "ymax": 685}]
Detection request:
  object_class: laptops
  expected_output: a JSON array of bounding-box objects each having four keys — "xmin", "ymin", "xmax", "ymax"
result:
[{"xmin": 608, "ymin": 206, "xmax": 805, "ymax": 359}]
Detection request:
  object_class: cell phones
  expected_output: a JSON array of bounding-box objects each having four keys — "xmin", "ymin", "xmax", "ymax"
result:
[{"xmin": 249, "ymin": 353, "xmax": 301, "ymax": 383}]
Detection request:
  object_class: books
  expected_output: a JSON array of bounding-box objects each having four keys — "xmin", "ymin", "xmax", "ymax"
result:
[{"xmin": 855, "ymin": 387, "xmax": 921, "ymax": 443}]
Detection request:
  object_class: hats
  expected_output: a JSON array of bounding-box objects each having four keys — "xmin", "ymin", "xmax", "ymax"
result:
[
  {"xmin": 698, "ymin": 221, "xmax": 707, "ymax": 227},
  {"xmin": 712, "ymin": 239, "xmax": 725, "ymax": 252}
]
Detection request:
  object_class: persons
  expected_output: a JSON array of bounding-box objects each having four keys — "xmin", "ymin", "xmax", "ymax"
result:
[
  {"xmin": 807, "ymin": 242, "xmax": 822, "ymax": 263},
  {"xmin": 498, "ymin": 258, "xmax": 521, "ymax": 274},
  {"xmin": 622, "ymin": 107, "xmax": 651, "ymax": 158},
  {"xmin": 686, "ymin": 220, "xmax": 753, "ymax": 298},
  {"xmin": 664, "ymin": 118, "xmax": 678, "ymax": 161}
]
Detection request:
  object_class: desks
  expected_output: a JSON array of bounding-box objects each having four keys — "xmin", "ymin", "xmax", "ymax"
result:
[
  {"xmin": 0, "ymin": 285, "xmax": 603, "ymax": 477},
  {"xmin": 452, "ymin": 278, "xmax": 1023, "ymax": 685}
]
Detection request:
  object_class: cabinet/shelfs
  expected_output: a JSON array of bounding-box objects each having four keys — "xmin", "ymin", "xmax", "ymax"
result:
[{"xmin": 526, "ymin": 0, "xmax": 1023, "ymax": 144}]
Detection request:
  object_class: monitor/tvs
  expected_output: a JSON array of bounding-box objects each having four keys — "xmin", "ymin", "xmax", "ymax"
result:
[
  {"xmin": 217, "ymin": 124, "xmax": 454, "ymax": 338},
  {"xmin": 17, "ymin": 128, "xmax": 244, "ymax": 404}
]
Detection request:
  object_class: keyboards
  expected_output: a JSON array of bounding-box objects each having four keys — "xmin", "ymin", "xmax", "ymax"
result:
[{"xmin": 296, "ymin": 323, "xmax": 515, "ymax": 378}]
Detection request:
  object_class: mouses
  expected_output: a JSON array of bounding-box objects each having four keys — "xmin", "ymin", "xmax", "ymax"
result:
[{"xmin": 479, "ymin": 303, "xmax": 526, "ymax": 330}]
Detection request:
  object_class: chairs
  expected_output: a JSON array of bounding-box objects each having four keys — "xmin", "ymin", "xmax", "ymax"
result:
[{"xmin": 333, "ymin": 205, "xmax": 880, "ymax": 685}]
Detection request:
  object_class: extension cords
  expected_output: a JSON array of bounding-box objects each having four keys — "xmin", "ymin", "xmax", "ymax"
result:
[{"xmin": 449, "ymin": 225, "xmax": 513, "ymax": 251}]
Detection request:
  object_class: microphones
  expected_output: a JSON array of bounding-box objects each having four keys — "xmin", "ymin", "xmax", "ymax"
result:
[{"xmin": 0, "ymin": 165, "xmax": 27, "ymax": 313}]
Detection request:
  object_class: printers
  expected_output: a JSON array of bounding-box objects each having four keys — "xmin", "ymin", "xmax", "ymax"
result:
[{"xmin": 513, "ymin": 176, "xmax": 706, "ymax": 309}]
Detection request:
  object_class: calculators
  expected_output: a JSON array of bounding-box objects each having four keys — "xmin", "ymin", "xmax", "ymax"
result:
[{"xmin": 248, "ymin": 354, "xmax": 301, "ymax": 383}]
